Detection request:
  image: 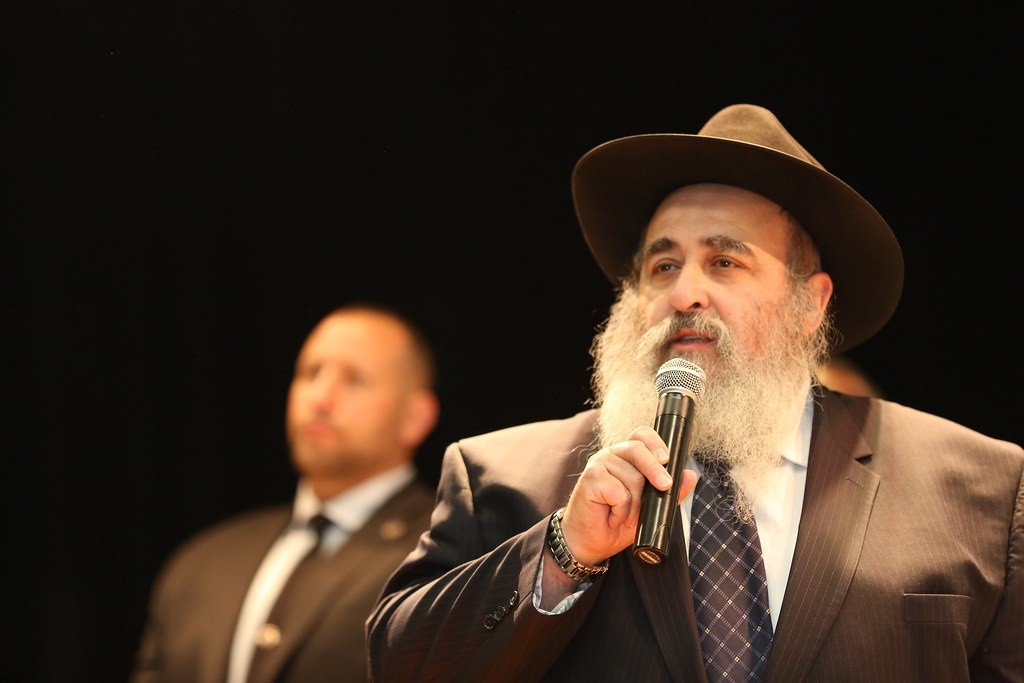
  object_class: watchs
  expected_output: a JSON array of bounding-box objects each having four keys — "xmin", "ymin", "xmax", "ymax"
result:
[{"xmin": 547, "ymin": 508, "xmax": 611, "ymax": 583}]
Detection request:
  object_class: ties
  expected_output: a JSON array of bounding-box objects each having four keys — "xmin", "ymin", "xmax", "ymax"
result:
[
  {"xmin": 687, "ymin": 441, "xmax": 774, "ymax": 682},
  {"xmin": 242, "ymin": 514, "xmax": 331, "ymax": 683}
]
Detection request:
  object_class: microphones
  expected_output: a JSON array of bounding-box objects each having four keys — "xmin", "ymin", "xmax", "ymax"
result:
[{"xmin": 632, "ymin": 357, "xmax": 708, "ymax": 569}]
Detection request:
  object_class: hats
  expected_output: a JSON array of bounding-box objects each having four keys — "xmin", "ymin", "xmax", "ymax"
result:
[{"xmin": 570, "ymin": 103, "xmax": 903, "ymax": 362}]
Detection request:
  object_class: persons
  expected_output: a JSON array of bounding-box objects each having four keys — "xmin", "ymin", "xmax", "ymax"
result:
[
  {"xmin": 134, "ymin": 309, "xmax": 439, "ymax": 683},
  {"xmin": 363, "ymin": 104, "xmax": 1024, "ymax": 683}
]
